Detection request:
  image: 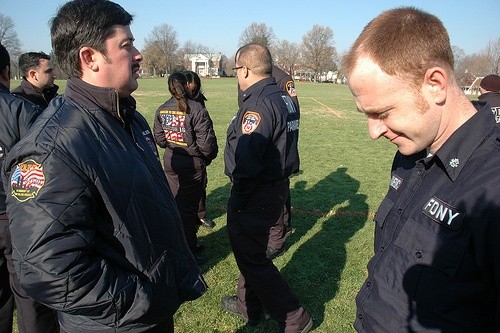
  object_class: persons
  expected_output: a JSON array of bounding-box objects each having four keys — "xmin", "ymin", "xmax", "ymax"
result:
[
  {"xmin": 233, "ymin": 42, "xmax": 301, "ymax": 261},
  {"xmin": 153, "ymin": 72, "xmax": 219, "ymax": 266},
  {"xmin": 219, "ymin": 42, "xmax": 314, "ymax": 333},
  {"xmin": 10, "ymin": 51, "xmax": 60, "ymax": 110},
  {"xmin": 340, "ymin": 7, "xmax": 500, "ymax": 333},
  {"xmin": 478, "ymin": 74, "xmax": 500, "ymax": 125},
  {"xmin": 0, "ymin": 0, "xmax": 210, "ymax": 333},
  {"xmin": 182, "ymin": 69, "xmax": 215, "ymax": 228},
  {"xmin": 0, "ymin": 42, "xmax": 61, "ymax": 333}
]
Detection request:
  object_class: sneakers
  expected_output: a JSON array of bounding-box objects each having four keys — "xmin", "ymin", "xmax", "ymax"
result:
[
  {"xmin": 198, "ymin": 218, "xmax": 215, "ymax": 228},
  {"xmin": 265, "ymin": 246, "xmax": 283, "ymax": 258},
  {"xmin": 275, "ymin": 306, "xmax": 313, "ymax": 333},
  {"xmin": 219, "ymin": 295, "xmax": 263, "ymax": 327}
]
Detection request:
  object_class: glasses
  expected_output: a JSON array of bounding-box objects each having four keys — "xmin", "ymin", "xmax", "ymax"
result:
[{"xmin": 233, "ymin": 66, "xmax": 250, "ymax": 70}]
